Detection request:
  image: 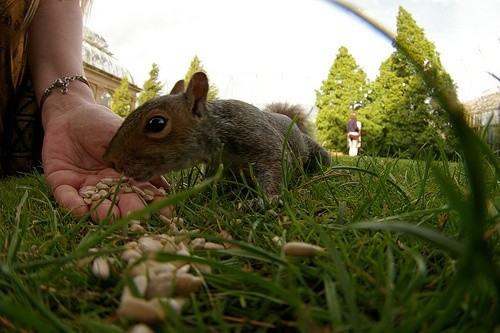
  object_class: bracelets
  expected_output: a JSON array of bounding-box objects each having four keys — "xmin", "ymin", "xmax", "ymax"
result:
[{"xmin": 39, "ymin": 74, "xmax": 91, "ymax": 119}]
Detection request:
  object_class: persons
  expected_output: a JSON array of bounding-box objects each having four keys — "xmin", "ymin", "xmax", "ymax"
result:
[
  {"xmin": 347, "ymin": 112, "xmax": 362, "ymax": 156},
  {"xmin": 0, "ymin": 0, "xmax": 175, "ymax": 224}
]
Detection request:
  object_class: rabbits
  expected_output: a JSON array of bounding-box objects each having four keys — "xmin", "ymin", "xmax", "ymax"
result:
[{"xmin": 101, "ymin": 71, "xmax": 330, "ymax": 214}]
